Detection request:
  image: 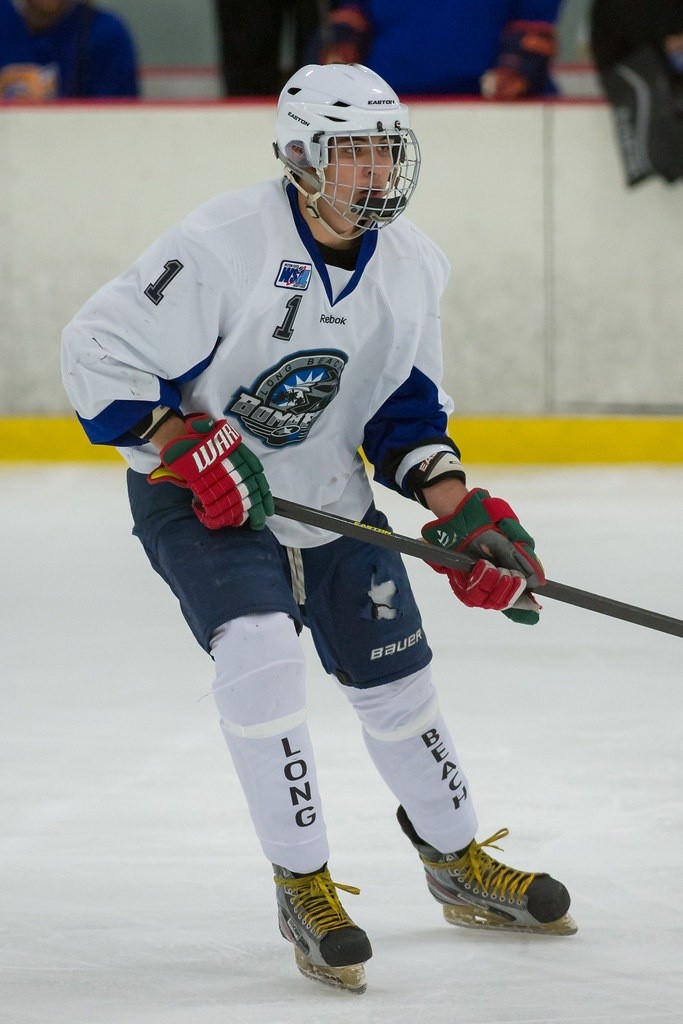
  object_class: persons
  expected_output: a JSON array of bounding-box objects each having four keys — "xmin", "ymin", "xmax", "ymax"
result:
[
  {"xmin": 322, "ymin": 0, "xmax": 682, "ymax": 186},
  {"xmin": 0, "ymin": 0, "xmax": 141, "ymax": 103},
  {"xmin": 56, "ymin": 59, "xmax": 574, "ymax": 971}
]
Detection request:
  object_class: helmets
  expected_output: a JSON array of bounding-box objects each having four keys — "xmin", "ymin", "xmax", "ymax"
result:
[{"xmin": 272, "ymin": 63, "xmax": 421, "ymax": 231}]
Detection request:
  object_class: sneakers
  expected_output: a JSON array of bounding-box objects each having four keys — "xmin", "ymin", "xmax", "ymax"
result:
[
  {"xmin": 272, "ymin": 864, "xmax": 372, "ymax": 994},
  {"xmin": 396, "ymin": 805, "xmax": 577, "ymax": 936}
]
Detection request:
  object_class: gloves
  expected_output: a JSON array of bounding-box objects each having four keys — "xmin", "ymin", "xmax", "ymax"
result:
[
  {"xmin": 147, "ymin": 412, "xmax": 274, "ymax": 531},
  {"xmin": 421, "ymin": 487, "xmax": 544, "ymax": 625}
]
